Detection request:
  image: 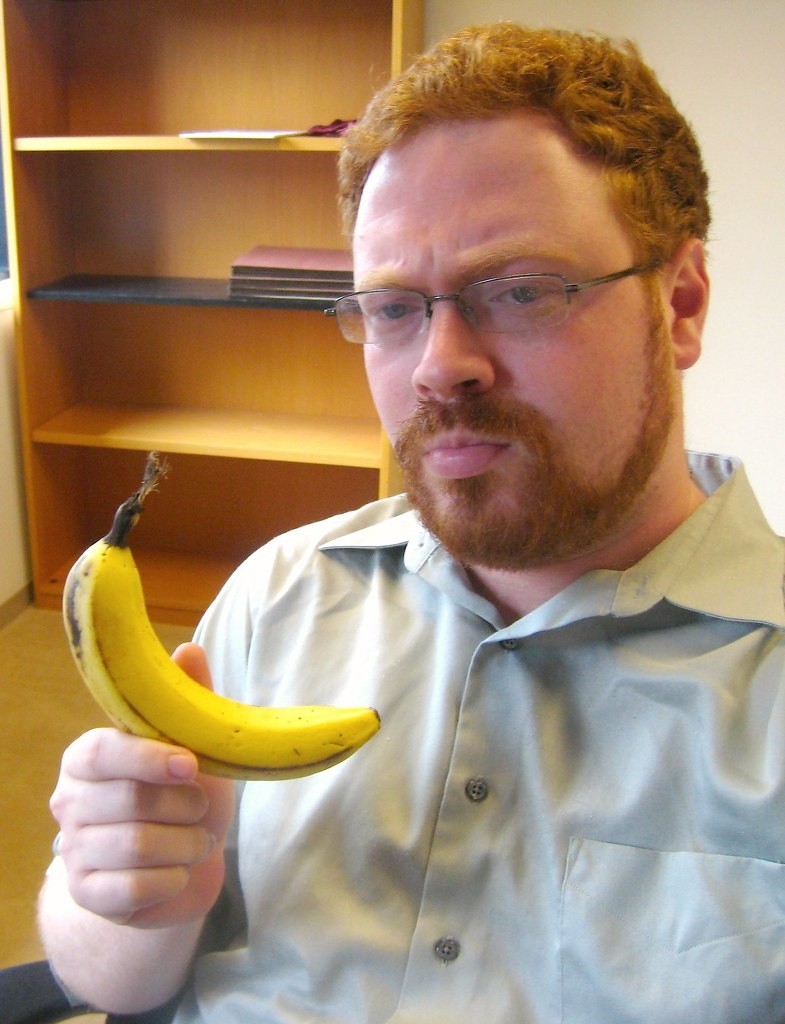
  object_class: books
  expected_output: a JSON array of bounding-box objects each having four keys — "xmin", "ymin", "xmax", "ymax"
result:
[{"xmin": 228, "ymin": 244, "xmax": 355, "ymax": 301}]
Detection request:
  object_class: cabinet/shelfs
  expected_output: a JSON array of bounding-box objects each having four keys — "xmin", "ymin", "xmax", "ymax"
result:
[{"xmin": 2, "ymin": 0, "xmax": 430, "ymax": 627}]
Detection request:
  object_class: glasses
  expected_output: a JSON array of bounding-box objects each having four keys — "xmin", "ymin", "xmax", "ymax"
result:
[{"xmin": 323, "ymin": 254, "xmax": 667, "ymax": 338}]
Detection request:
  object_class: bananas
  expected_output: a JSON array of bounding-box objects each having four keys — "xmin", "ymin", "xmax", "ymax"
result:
[{"xmin": 61, "ymin": 451, "xmax": 379, "ymax": 781}]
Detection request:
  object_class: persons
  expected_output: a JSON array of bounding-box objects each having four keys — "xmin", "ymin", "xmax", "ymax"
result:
[{"xmin": 37, "ymin": 19, "xmax": 785, "ymax": 1024}]
